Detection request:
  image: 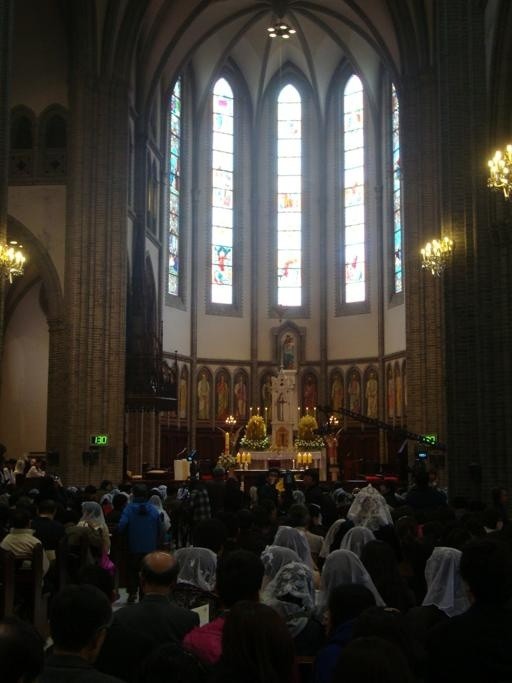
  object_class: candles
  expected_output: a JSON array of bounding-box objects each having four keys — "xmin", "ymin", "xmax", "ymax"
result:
[
  {"xmin": 297, "ymin": 406, "xmax": 317, "ymax": 421},
  {"xmin": 296, "ymin": 452, "xmax": 314, "ymax": 464},
  {"xmin": 248, "ymin": 406, "xmax": 268, "ymax": 427},
  {"xmin": 225, "ymin": 431, "xmax": 229, "ymax": 453},
  {"xmin": 237, "ymin": 451, "xmax": 252, "ymax": 464}
]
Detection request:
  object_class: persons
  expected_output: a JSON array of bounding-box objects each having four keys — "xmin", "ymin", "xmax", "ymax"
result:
[
  {"xmin": 178, "ymin": 373, "xmax": 186, "ymax": 418},
  {"xmin": 364, "ymin": 370, "xmax": 377, "ymax": 419},
  {"xmin": 261, "ymin": 374, "xmax": 272, "ymax": 408},
  {"xmin": 330, "ymin": 374, "xmax": 343, "ymax": 411},
  {"xmin": 303, "ymin": 375, "xmax": 317, "ymax": 414},
  {"xmin": 386, "ymin": 358, "xmax": 407, "ymax": 419},
  {"xmin": 0, "ymin": 445, "xmax": 512, "ymax": 683},
  {"xmin": 234, "ymin": 375, "xmax": 246, "ymax": 417},
  {"xmin": 216, "ymin": 374, "xmax": 228, "ymax": 417},
  {"xmin": 347, "ymin": 370, "xmax": 360, "ymax": 414},
  {"xmin": 197, "ymin": 372, "xmax": 210, "ymax": 420}
]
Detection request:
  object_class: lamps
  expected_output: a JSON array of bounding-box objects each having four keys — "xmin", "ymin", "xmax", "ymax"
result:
[
  {"xmin": 420, "ymin": 236, "xmax": 454, "ymax": 279},
  {"xmin": 0, "ymin": 239, "xmax": 27, "ymax": 285},
  {"xmin": 486, "ymin": 144, "xmax": 511, "ymax": 202},
  {"xmin": 265, "ymin": 7, "xmax": 295, "ymax": 41}
]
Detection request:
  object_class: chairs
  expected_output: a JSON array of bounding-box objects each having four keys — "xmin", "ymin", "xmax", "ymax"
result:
[{"xmin": 361, "ymin": 462, "xmax": 399, "ymax": 490}]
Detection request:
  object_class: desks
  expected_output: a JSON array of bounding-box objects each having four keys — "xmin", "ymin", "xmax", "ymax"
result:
[{"xmin": 235, "ymin": 469, "xmax": 306, "ymax": 490}]
